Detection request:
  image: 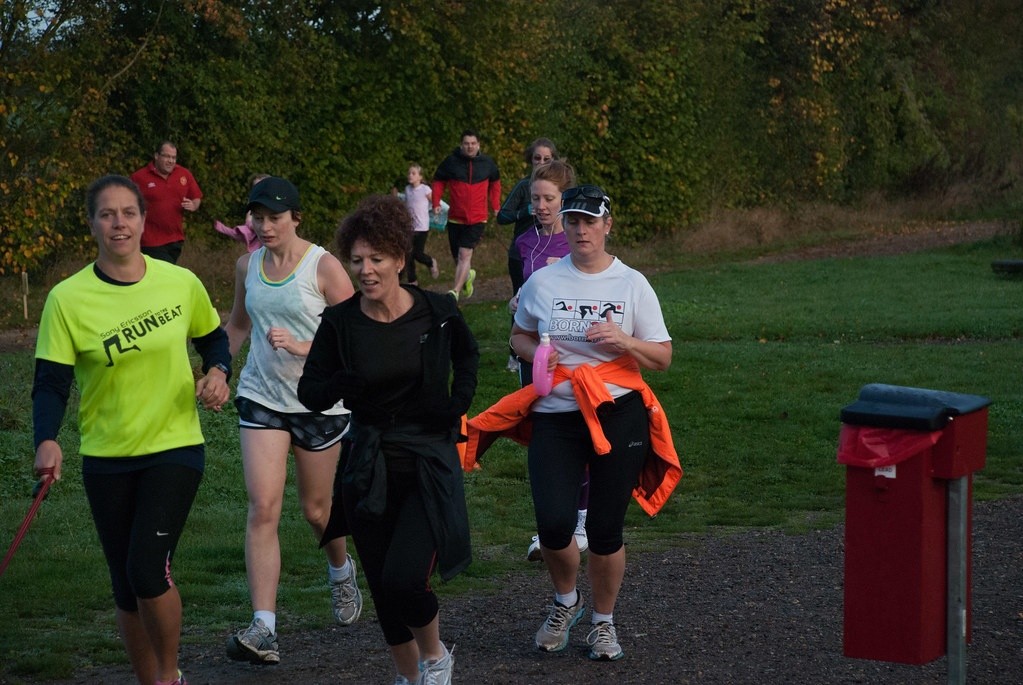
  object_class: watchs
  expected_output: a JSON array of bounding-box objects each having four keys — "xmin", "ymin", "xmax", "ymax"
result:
[{"xmin": 216, "ymin": 363, "xmax": 228, "ymax": 375}]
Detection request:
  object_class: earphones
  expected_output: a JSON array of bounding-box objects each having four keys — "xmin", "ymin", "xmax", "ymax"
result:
[
  {"xmin": 478, "ymin": 145, "xmax": 480, "ymax": 149},
  {"xmin": 461, "ymin": 145, "xmax": 462, "ymax": 149}
]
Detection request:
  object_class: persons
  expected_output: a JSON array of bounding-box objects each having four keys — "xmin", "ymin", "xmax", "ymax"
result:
[
  {"xmin": 508, "ymin": 186, "xmax": 673, "ymax": 661},
  {"xmin": 128, "ymin": 140, "xmax": 202, "ymax": 265},
  {"xmin": 224, "ymin": 176, "xmax": 358, "ymax": 666},
  {"xmin": 213, "ymin": 172, "xmax": 269, "ymax": 252},
  {"xmin": 391, "ymin": 162, "xmax": 440, "ymax": 286},
  {"xmin": 503, "ymin": 159, "xmax": 589, "ymax": 562},
  {"xmin": 31, "ymin": 174, "xmax": 231, "ymax": 685},
  {"xmin": 297, "ymin": 196, "xmax": 478, "ymax": 685},
  {"xmin": 495, "ymin": 138, "xmax": 557, "ymax": 371},
  {"xmin": 431, "ymin": 130, "xmax": 501, "ymax": 302}
]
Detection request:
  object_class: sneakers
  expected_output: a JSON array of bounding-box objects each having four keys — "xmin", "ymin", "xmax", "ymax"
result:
[
  {"xmin": 328, "ymin": 554, "xmax": 362, "ymax": 628},
  {"xmin": 585, "ymin": 621, "xmax": 624, "ymax": 661},
  {"xmin": 448, "ymin": 289, "xmax": 459, "ymax": 305},
  {"xmin": 535, "ymin": 588, "xmax": 585, "ymax": 652},
  {"xmin": 575, "ymin": 509, "xmax": 588, "ymax": 552},
  {"xmin": 461, "ymin": 269, "xmax": 476, "ymax": 299},
  {"xmin": 225, "ymin": 618, "xmax": 280, "ymax": 664},
  {"xmin": 527, "ymin": 535, "xmax": 542, "ymax": 561}
]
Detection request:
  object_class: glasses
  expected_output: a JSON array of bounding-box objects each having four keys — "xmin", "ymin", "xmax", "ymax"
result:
[
  {"xmin": 561, "ymin": 186, "xmax": 608, "ymax": 210},
  {"xmin": 532, "ymin": 154, "xmax": 551, "ymax": 163}
]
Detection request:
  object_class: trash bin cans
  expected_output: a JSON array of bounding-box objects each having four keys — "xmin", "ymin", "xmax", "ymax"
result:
[{"xmin": 838, "ymin": 383, "xmax": 994, "ymax": 667}]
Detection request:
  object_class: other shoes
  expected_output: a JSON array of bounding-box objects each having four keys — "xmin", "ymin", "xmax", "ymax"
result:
[
  {"xmin": 410, "ymin": 280, "xmax": 419, "ymax": 288},
  {"xmin": 394, "ymin": 674, "xmax": 410, "ymax": 684},
  {"xmin": 171, "ymin": 669, "xmax": 186, "ymax": 685},
  {"xmin": 507, "ymin": 355, "xmax": 520, "ymax": 373},
  {"xmin": 429, "ymin": 259, "xmax": 438, "ymax": 279},
  {"xmin": 417, "ymin": 640, "xmax": 456, "ymax": 685}
]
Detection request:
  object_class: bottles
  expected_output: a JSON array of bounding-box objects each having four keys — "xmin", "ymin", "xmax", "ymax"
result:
[{"xmin": 532, "ymin": 333, "xmax": 555, "ymax": 396}]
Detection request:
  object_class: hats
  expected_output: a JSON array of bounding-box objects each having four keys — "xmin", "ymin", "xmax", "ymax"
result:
[
  {"xmin": 244, "ymin": 176, "xmax": 301, "ymax": 214},
  {"xmin": 556, "ymin": 187, "xmax": 610, "ymax": 217}
]
